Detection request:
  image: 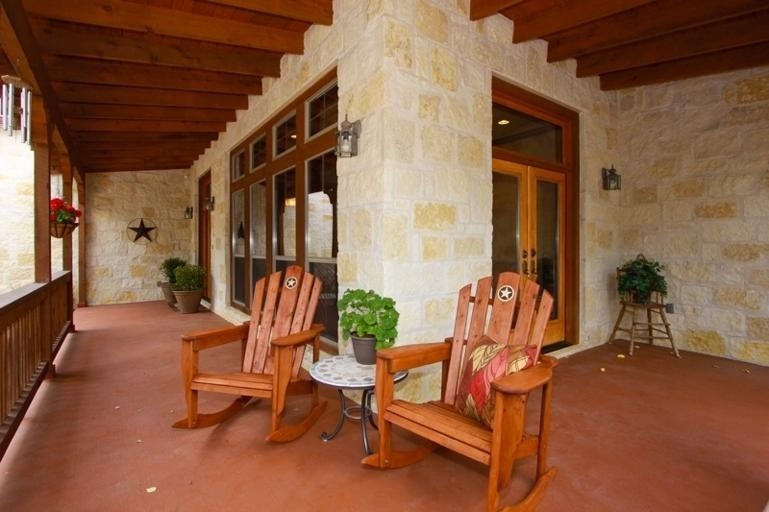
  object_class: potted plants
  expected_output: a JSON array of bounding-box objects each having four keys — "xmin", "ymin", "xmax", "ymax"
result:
[
  {"xmin": 172, "ymin": 266, "xmax": 207, "ymax": 314},
  {"xmin": 616, "ymin": 254, "xmax": 667, "ymax": 302},
  {"xmin": 335, "ymin": 287, "xmax": 399, "ymax": 365},
  {"xmin": 158, "ymin": 258, "xmax": 186, "ymax": 305}
]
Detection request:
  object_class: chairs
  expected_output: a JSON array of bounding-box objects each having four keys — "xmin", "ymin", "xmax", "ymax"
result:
[
  {"xmin": 362, "ymin": 270, "xmax": 557, "ymax": 512},
  {"xmin": 609, "ymin": 266, "xmax": 681, "ymax": 359},
  {"xmin": 170, "ymin": 266, "xmax": 328, "ymax": 445}
]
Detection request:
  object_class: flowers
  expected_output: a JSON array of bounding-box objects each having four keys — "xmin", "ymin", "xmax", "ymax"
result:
[{"xmin": 48, "ymin": 197, "xmax": 83, "ymax": 224}]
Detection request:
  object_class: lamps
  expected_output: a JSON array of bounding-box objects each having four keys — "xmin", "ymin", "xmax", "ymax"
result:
[
  {"xmin": 335, "ymin": 114, "xmax": 360, "ymax": 157},
  {"xmin": 203, "ymin": 195, "xmax": 215, "ymax": 211},
  {"xmin": 184, "ymin": 208, "xmax": 193, "ymax": 219},
  {"xmin": 601, "ymin": 165, "xmax": 622, "ymax": 190}
]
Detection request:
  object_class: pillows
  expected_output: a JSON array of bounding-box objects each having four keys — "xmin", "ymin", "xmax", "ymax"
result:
[{"xmin": 454, "ymin": 335, "xmax": 537, "ymax": 432}]
